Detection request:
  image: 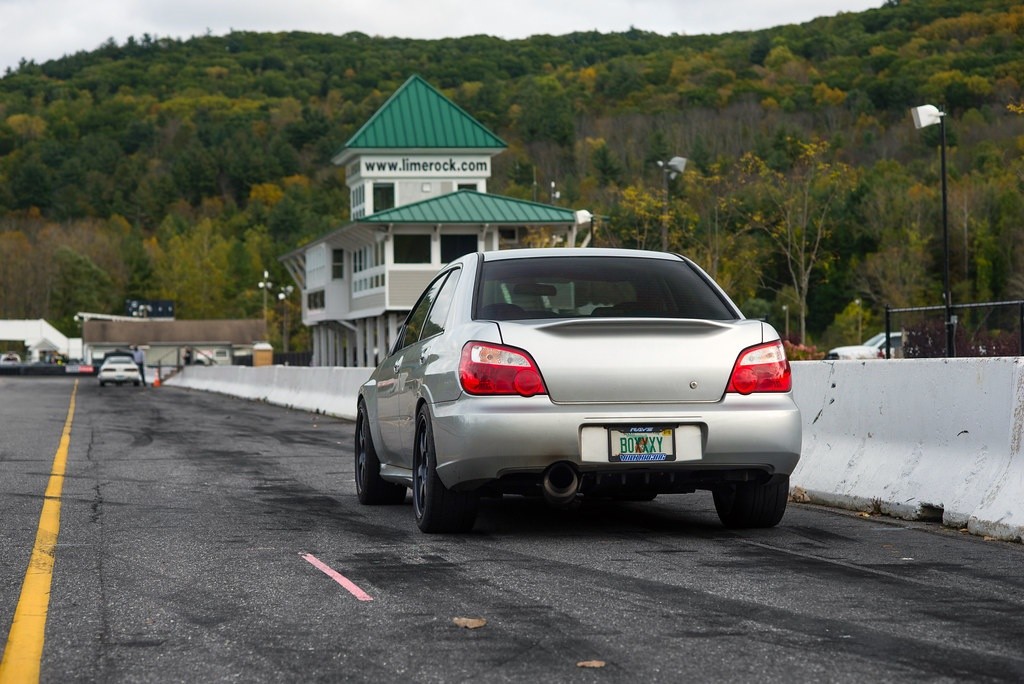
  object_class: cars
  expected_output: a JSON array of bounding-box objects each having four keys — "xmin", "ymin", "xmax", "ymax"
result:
[
  {"xmin": 1, "ymin": 353, "xmax": 21, "ymax": 367},
  {"xmin": 352, "ymin": 245, "xmax": 805, "ymax": 534},
  {"xmin": 97, "ymin": 355, "xmax": 142, "ymax": 387},
  {"xmin": 828, "ymin": 330, "xmax": 907, "ymax": 360}
]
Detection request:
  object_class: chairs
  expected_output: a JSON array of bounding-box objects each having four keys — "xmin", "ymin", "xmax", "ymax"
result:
[
  {"xmin": 482, "ymin": 303, "xmax": 526, "ymax": 320},
  {"xmin": 588, "ymin": 302, "xmax": 638, "ymax": 316}
]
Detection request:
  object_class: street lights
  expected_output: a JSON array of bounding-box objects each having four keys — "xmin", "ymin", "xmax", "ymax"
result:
[
  {"xmin": 656, "ymin": 156, "xmax": 689, "ymax": 255},
  {"xmin": 279, "ymin": 284, "xmax": 295, "ymax": 354},
  {"xmin": 912, "ymin": 104, "xmax": 958, "ymax": 359},
  {"xmin": 258, "ymin": 270, "xmax": 273, "ymax": 341}
]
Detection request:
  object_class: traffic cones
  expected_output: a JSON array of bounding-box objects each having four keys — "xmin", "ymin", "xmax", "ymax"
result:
[{"xmin": 154, "ymin": 369, "xmax": 161, "ymax": 387}]
